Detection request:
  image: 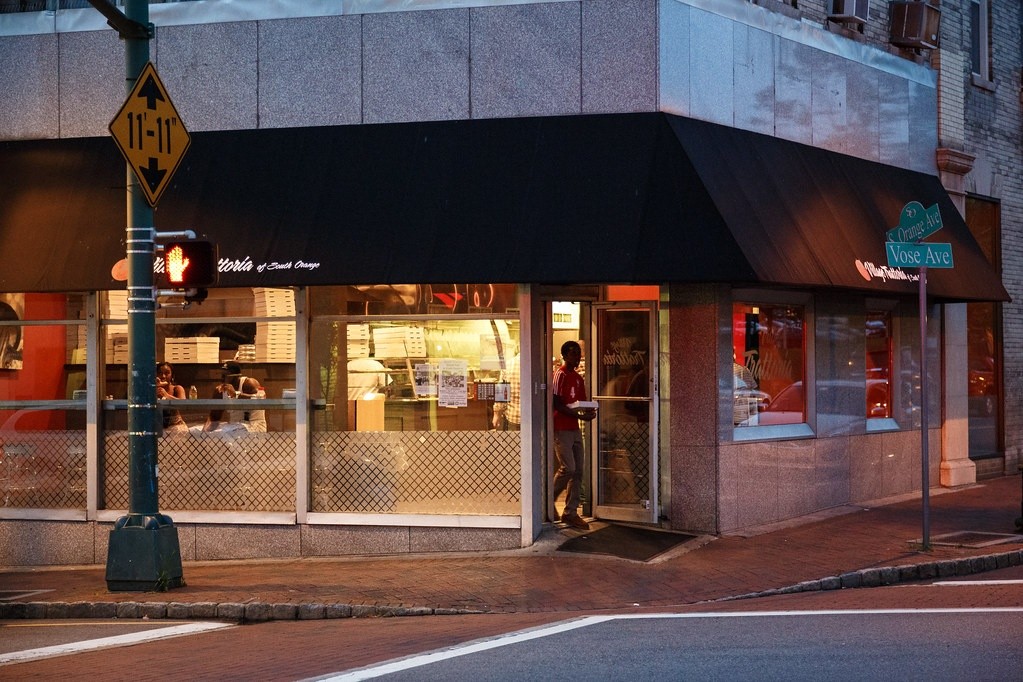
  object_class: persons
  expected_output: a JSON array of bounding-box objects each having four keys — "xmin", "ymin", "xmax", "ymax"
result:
[
  {"xmin": 733, "ymin": 348, "xmax": 760, "ymax": 429},
  {"xmin": 346, "ymin": 355, "xmax": 393, "ymax": 400},
  {"xmin": 157, "ymin": 361, "xmax": 190, "ymax": 434},
  {"xmin": 600, "ymin": 351, "xmax": 650, "ymax": 509},
  {"xmin": 203, "ymin": 361, "xmax": 269, "ymax": 436},
  {"xmin": 552, "ymin": 341, "xmax": 597, "ymax": 530}
]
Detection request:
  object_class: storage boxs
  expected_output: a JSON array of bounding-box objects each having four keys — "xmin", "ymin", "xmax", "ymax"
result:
[{"xmin": 68, "ymin": 290, "xmax": 297, "ymax": 363}]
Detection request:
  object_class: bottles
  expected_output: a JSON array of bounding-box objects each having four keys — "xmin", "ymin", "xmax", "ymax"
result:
[{"xmin": 189, "ymin": 386, "xmax": 198, "ymax": 400}]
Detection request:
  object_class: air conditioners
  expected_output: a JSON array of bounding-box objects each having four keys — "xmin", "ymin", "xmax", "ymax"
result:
[
  {"xmin": 890, "ymin": 0, "xmax": 941, "ymax": 51},
  {"xmin": 826, "ymin": 0, "xmax": 870, "ymax": 24}
]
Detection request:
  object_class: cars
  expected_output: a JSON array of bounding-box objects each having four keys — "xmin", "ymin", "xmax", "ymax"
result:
[
  {"xmin": 735, "ymin": 374, "xmax": 922, "ymax": 427},
  {"xmin": 732, "ymin": 361, "xmax": 776, "ymax": 422}
]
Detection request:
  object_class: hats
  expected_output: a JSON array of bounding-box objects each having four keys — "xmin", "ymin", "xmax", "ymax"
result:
[{"xmin": 220, "ymin": 361, "xmax": 241, "ymax": 376}]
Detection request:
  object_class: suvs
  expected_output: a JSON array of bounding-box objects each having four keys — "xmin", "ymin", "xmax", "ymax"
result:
[{"xmin": 924, "ymin": 345, "xmax": 998, "ymax": 419}]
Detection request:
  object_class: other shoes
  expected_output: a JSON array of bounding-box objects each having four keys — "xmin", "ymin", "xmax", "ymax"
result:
[
  {"xmin": 561, "ymin": 515, "xmax": 589, "ymax": 531},
  {"xmin": 546, "ymin": 502, "xmax": 561, "ymax": 521}
]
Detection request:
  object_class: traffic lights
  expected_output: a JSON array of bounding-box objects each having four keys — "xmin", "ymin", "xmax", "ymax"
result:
[{"xmin": 156, "ymin": 239, "xmax": 221, "ymax": 290}]
